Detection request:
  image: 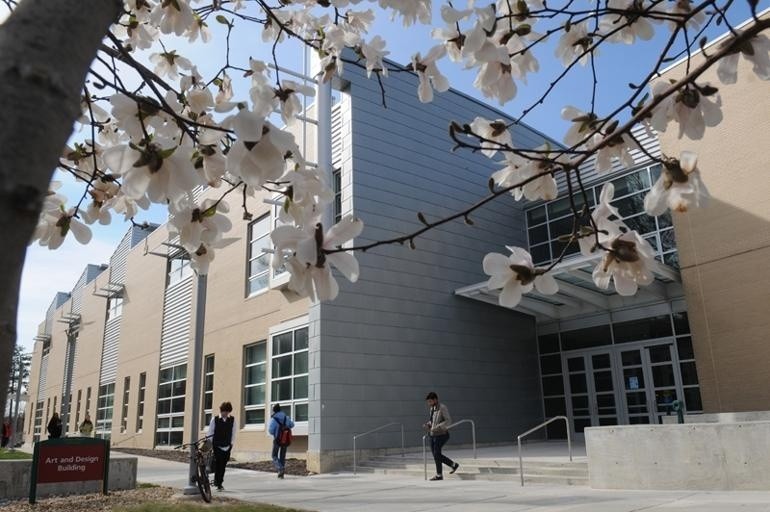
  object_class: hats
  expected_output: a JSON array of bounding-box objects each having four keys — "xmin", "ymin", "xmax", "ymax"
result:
[{"xmin": 272, "ymin": 402, "xmax": 281, "ymax": 412}]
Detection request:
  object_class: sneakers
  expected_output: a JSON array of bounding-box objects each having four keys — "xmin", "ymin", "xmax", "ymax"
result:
[
  {"xmin": 449, "ymin": 462, "xmax": 459, "ymax": 474},
  {"xmin": 429, "ymin": 475, "xmax": 444, "ymax": 481}
]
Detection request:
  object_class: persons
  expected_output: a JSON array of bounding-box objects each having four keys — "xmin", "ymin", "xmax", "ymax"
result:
[
  {"xmin": 0, "ymin": 422, "xmax": 6, "ymax": 447},
  {"xmin": 268, "ymin": 403, "xmax": 295, "ymax": 480},
  {"xmin": 203, "ymin": 402, "xmax": 237, "ymax": 491},
  {"xmin": 78, "ymin": 414, "xmax": 93, "ymax": 438},
  {"xmin": 55, "ymin": 420, "xmax": 62, "ymax": 439},
  {"xmin": 47, "ymin": 413, "xmax": 58, "ymax": 439},
  {"xmin": 3, "ymin": 420, "xmax": 12, "ymax": 447},
  {"xmin": 422, "ymin": 391, "xmax": 459, "ymax": 481}
]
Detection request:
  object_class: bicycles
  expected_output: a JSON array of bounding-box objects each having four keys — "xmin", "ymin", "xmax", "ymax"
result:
[{"xmin": 173, "ymin": 434, "xmax": 216, "ymax": 503}]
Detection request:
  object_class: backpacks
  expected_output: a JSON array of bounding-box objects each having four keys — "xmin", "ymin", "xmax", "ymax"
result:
[{"xmin": 272, "ymin": 415, "xmax": 292, "ymax": 447}]
